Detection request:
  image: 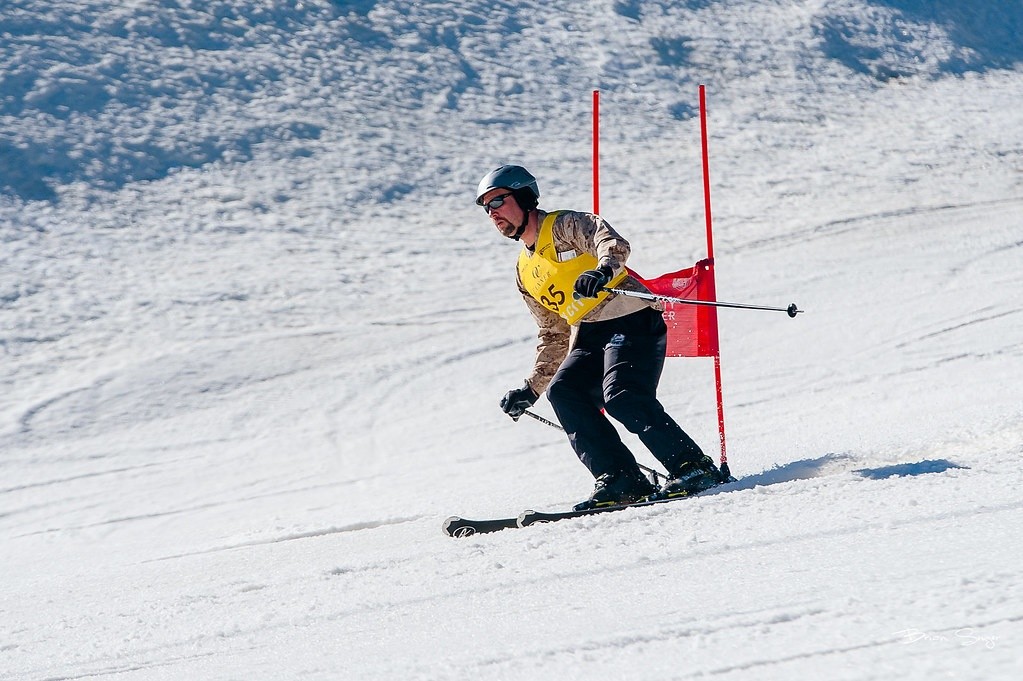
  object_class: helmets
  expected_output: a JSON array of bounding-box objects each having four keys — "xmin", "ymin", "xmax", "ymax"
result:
[{"xmin": 475, "ymin": 165, "xmax": 540, "ymax": 212}]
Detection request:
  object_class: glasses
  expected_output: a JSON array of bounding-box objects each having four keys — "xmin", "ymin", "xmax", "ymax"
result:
[{"xmin": 483, "ymin": 191, "xmax": 514, "ymax": 213}]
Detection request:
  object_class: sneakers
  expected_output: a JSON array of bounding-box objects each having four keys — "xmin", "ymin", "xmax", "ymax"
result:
[
  {"xmin": 574, "ymin": 469, "xmax": 655, "ymax": 510},
  {"xmin": 647, "ymin": 458, "xmax": 724, "ymax": 500}
]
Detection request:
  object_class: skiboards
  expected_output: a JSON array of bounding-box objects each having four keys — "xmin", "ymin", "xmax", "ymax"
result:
[{"xmin": 441, "ymin": 495, "xmax": 690, "ymax": 538}]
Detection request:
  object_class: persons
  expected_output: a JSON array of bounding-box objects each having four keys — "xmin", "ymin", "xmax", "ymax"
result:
[{"xmin": 475, "ymin": 165, "xmax": 720, "ymax": 511}]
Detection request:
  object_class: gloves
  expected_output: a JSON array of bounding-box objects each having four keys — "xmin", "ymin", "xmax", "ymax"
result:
[
  {"xmin": 500, "ymin": 379, "xmax": 539, "ymax": 422},
  {"xmin": 574, "ymin": 265, "xmax": 614, "ymax": 298}
]
